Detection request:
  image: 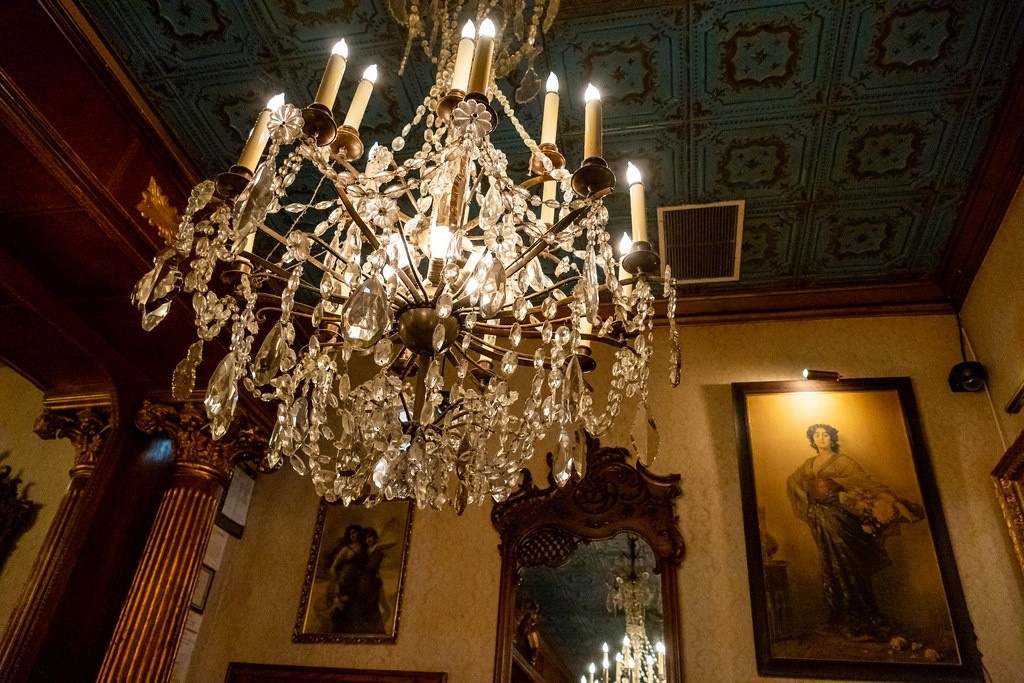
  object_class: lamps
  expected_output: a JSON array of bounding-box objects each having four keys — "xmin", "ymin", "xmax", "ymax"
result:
[{"xmin": 131, "ymin": 1, "xmax": 681, "ymax": 515}]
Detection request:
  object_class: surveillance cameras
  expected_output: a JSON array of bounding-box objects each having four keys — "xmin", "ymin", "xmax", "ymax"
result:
[{"xmin": 948, "ymin": 360, "xmax": 987, "ymax": 392}]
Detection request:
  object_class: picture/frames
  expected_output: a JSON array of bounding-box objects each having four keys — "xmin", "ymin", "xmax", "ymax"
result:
[
  {"xmin": 292, "ymin": 496, "xmax": 416, "ymax": 645},
  {"xmin": 731, "ymin": 377, "xmax": 985, "ymax": 683}
]
